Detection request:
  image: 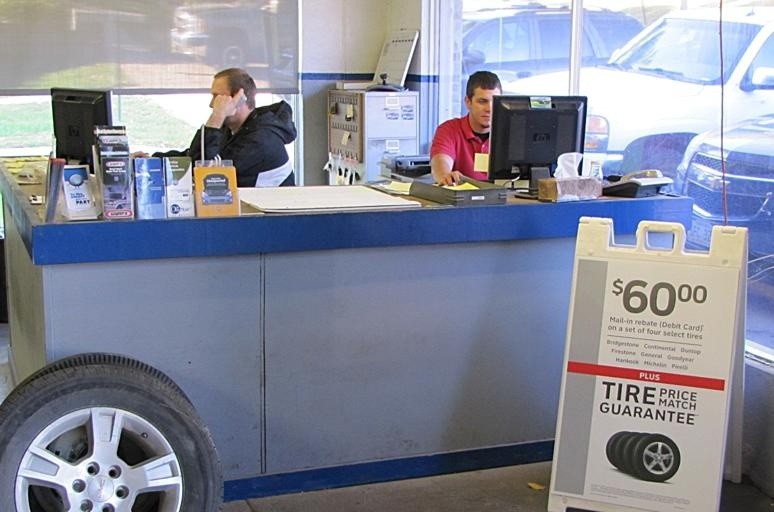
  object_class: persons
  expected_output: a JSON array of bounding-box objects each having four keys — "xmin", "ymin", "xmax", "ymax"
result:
[
  {"xmin": 133, "ymin": 68, "xmax": 297, "ymax": 186},
  {"xmin": 429, "ymin": 71, "xmax": 506, "ymax": 187}
]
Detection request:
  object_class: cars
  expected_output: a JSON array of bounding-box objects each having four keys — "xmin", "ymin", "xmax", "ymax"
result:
[{"xmin": 170, "ymin": 5, "xmax": 294, "ymax": 71}]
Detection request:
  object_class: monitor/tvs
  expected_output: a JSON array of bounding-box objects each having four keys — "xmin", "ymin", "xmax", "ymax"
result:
[
  {"xmin": 51, "ymin": 87, "xmax": 112, "ymax": 173},
  {"xmin": 488, "ymin": 94, "xmax": 587, "ymax": 199}
]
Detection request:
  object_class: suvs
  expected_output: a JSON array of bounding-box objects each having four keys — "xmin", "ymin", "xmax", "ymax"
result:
[
  {"xmin": 675, "ymin": 115, "xmax": 774, "ymax": 299},
  {"xmin": 459, "ymin": 5, "xmax": 646, "ymax": 119},
  {"xmin": 495, "ymin": 8, "xmax": 774, "ymax": 184}
]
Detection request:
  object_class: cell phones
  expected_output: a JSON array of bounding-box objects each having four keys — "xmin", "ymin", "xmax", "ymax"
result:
[{"xmin": 235, "ymin": 94, "xmax": 247, "ymax": 108}]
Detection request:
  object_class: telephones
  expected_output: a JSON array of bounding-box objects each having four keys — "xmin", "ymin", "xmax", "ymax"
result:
[{"xmin": 603, "ymin": 168, "xmax": 673, "ymax": 197}]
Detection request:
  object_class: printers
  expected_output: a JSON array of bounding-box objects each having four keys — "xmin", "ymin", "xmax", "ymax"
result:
[{"xmin": 387, "ymin": 153, "xmax": 431, "ymax": 179}]
